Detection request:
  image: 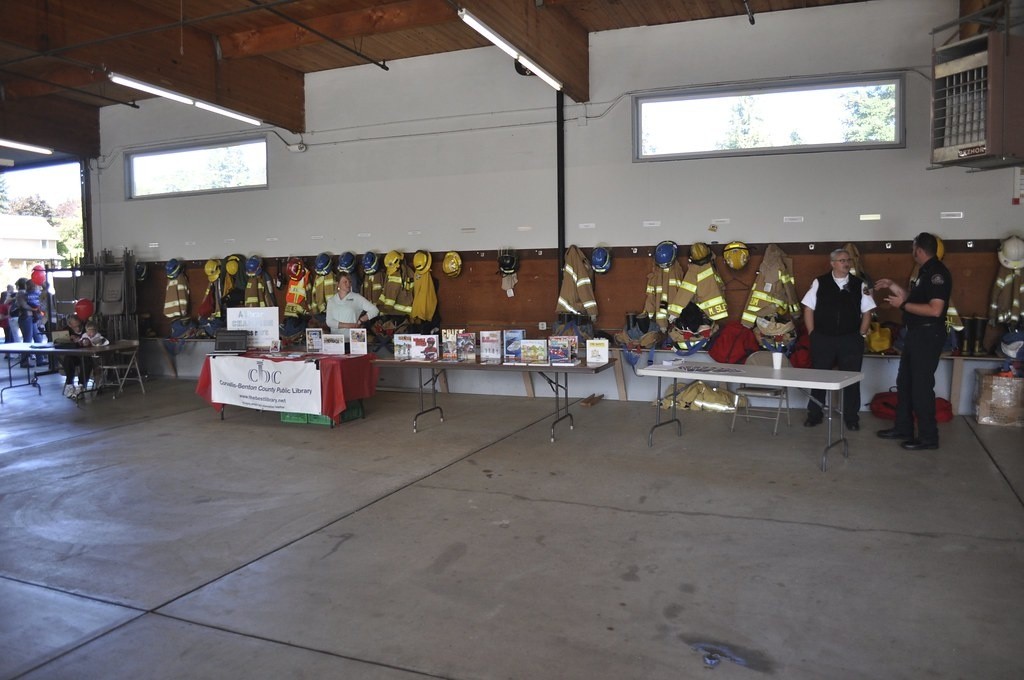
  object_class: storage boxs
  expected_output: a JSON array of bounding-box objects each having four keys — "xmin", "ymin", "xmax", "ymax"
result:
[
  {"xmin": 339, "ymin": 399, "xmax": 364, "ymax": 423},
  {"xmin": 308, "ymin": 415, "xmax": 335, "ymax": 426},
  {"xmin": 974, "ymin": 368, "xmax": 1024, "ymax": 428},
  {"xmin": 280, "ymin": 412, "xmax": 307, "ymax": 423}
]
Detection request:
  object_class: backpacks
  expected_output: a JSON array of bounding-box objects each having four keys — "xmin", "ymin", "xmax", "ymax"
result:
[{"xmin": 10, "ymin": 303, "xmax": 20, "ymax": 317}]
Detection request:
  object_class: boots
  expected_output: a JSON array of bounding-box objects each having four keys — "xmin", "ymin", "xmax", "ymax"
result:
[
  {"xmin": 960, "ymin": 317, "xmax": 973, "ymax": 355},
  {"xmin": 973, "ymin": 317, "xmax": 988, "ymax": 355},
  {"xmin": 143, "ymin": 314, "xmax": 156, "ymax": 337}
]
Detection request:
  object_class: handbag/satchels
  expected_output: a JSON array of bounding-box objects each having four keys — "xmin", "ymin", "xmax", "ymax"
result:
[
  {"xmin": 1001, "ymin": 331, "xmax": 1024, "ymax": 377},
  {"xmin": 871, "ymin": 386, "xmax": 953, "ymax": 424},
  {"xmin": 555, "ymin": 317, "xmax": 892, "ymax": 369}
]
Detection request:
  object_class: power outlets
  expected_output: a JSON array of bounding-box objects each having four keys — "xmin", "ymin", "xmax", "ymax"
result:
[{"xmin": 539, "ymin": 322, "xmax": 546, "ymax": 330}]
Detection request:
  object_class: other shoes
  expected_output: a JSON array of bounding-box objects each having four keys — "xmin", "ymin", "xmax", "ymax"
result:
[
  {"xmin": 847, "ymin": 422, "xmax": 859, "ymax": 431},
  {"xmin": 39, "ymin": 324, "xmax": 46, "ymax": 333},
  {"xmin": 4, "ymin": 353, "xmax": 50, "ymax": 367},
  {"xmin": 804, "ymin": 416, "xmax": 822, "ymax": 427}
]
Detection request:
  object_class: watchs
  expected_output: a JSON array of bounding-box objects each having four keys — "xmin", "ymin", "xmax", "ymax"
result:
[{"xmin": 900, "ymin": 302, "xmax": 906, "ymax": 310}]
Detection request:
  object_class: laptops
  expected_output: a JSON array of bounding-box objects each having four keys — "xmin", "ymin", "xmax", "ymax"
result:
[
  {"xmin": 52, "ymin": 331, "xmax": 71, "ymax": 342},
  {"xmin": 205, "ymin": 330, "xmax": 248, "ymax": 355}
]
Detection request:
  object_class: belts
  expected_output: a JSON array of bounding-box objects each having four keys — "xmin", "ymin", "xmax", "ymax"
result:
[{"xmin": 909, "ymin": 322, "xmax": 936, "ymax": 327}]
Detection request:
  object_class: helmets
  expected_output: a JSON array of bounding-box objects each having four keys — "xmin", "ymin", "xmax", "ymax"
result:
[
  {"xmin": 427, "ymin": 337, "xmax": 435, "ymax": 343},
  {"xmin": 286, "ymin": 250, "xmax": 463, "ymax": 281},
  {"xmin": 998, "ymin": 236, "xmax": 1024, "ymax": 269},
  {"xmin": 498, "ymin": 252, "xmax": 518, "ymax": 275},
  {"xmin": 654, "ymin": 241, "xmax": 750, "ymax": 271},
  {"xmin": 591, "ymin": 247, "xmax": 611, "ymax": 274},
  {"xmin": 135, "ymin": 265, "xmax": 148, "ymax": 282},
  {"xmin": 204, "ymin": 256, "xmax": 264, "ymax": 282},
  {"xmin": 165, "ymin": 259, "xmax": 181, "ymax": 279}
]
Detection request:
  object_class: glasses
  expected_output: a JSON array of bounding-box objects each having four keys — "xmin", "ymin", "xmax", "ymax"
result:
[{"xmin": 832, "ymin": 258, "xmax": 851, "ymax": 263}]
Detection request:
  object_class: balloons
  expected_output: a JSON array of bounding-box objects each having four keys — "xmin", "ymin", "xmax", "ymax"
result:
[
  {"xmin": 76, "ymin": 299, "xmax": 94, "ymax": 320},
  {"xmin": 32, "ymin": 265, "xmax": 46, "ymax": 285}
]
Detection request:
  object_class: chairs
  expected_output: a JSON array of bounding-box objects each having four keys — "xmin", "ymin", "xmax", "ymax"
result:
[
  {"xmin": 730, "ymin": 351, "xmax": 791, "ymax": 436},
  {"xmin": 53, "ymin": 247, "xmax": 146, "ymax": 396}
]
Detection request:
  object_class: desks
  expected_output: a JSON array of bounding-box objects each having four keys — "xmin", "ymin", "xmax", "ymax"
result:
[
  {"xmin": 143, "ymin": 337, "xmax": 1024, "ymax": 473},
  {"xmin": 0, "ymin": 343, "xmax": 138, "ymax": 407}
]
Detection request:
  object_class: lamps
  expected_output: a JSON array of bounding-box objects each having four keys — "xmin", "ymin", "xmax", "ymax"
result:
[
  {"xmin": 108, "ymin": 71, "xmax": 262, "ymax": 126},
  {"xmin": 0, "ymin": 139, "xmax": 54, "ymax": 154},
  {"xmin": 458, "ymin": 8, "xmax": 565, "ymax": 91}
]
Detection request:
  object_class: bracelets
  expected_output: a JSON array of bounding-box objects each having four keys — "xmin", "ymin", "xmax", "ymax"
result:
[
  {"xmin": 359, "ymin": 319, "xmax": 363, "ymax": 323},
  {"xmin": 861, "ymin": 334, "xmax": 866, "ymax": 338}
]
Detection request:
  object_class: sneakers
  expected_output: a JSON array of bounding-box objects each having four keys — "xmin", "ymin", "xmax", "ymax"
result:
[{"xmin": 65, "ymin": 382, "xmax": 84, "ymax": 399}]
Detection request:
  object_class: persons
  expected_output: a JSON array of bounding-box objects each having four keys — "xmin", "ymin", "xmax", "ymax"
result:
[
  {"xmin": 326, "ymin": 272, "xmax": 379, "ymax": 350},
  {"xmin": 0, "ymin": 278, "xmax": 49, "ymax": 367},
  {"xmin": 801, "ymin": 250, "xmax": 877, "ymax": 430},
  {"xmin": 875, "ymin": 233, "xmax": 952, "ymax": 450},
  {"xmin": 64, "ymin": 314, "xmax": 109, "ymax": 398}
]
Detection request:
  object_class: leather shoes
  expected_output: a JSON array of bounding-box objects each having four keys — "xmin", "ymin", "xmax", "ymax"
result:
[
  {"xmin": 901, "ymin": 439, "xmax": 937, "ymax": 450},
  {"xmin": 878, "ymin": 429, "xmax": 914, "ymax": 440}
]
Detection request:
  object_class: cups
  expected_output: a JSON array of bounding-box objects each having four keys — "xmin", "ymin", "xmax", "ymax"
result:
[{"xmin": 773, "ymin": 353, "xmax": 782, "ymax": 369}]
